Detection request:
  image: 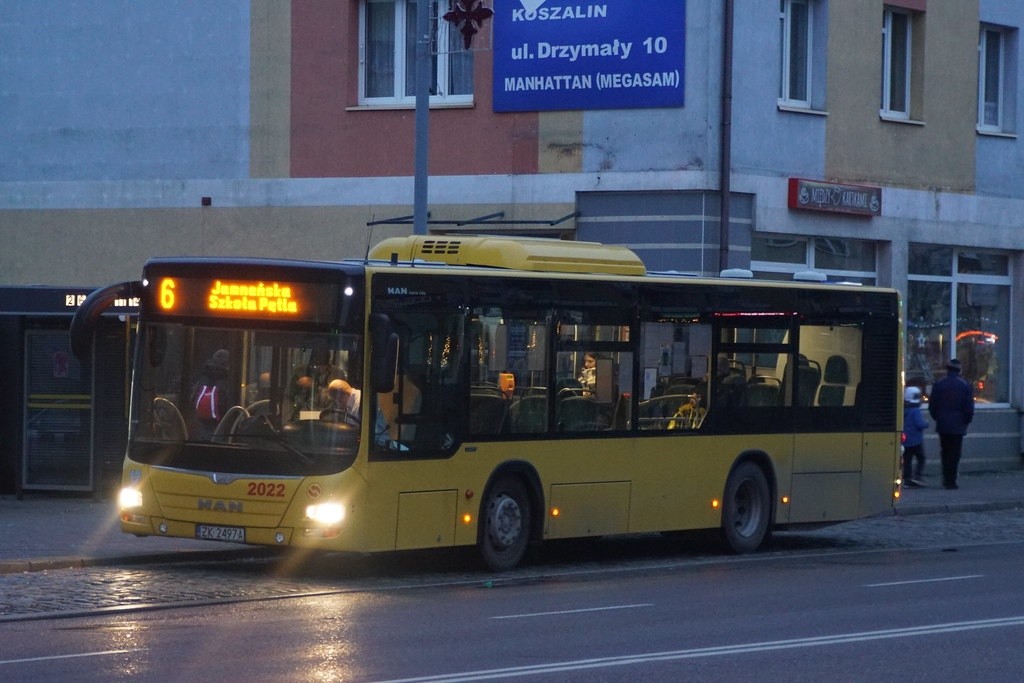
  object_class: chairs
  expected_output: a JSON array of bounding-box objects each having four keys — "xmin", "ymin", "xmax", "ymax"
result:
[{"xmin": 378, "ymin": 353, "xmax": 850, "ymax": 446}]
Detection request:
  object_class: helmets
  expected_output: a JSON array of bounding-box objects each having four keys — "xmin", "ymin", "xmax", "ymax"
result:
[{"xmin": 904, "ymin": 387, "xmax": 922, "ymax": 404}]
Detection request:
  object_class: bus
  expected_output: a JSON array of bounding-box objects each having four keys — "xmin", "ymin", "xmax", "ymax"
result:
[{"xmin": 67, "ymin": 229, "xmax": 907, "ymax": 572}]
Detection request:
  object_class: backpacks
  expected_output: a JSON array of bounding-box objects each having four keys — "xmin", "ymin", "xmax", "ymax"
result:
[{"xmin": 194, "ymin": 384, "xmax": 221, "ymax": 422}]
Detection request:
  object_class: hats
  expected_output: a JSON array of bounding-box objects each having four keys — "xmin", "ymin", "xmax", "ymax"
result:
[{"xmin": 948, "ymin": 360, "xmax": 963, "ymax": 372}]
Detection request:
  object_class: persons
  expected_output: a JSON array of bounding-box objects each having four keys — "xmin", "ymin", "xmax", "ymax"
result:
[
  {"xmin": 576, "ymin": 348, "xmax": 600, "ymax": 396},
  {"xmin": 929, "ymin": 358, "xmax": 974, "ymax": 489},
  {"xmin": 690, "ymin": 355, "xmax": 743, "ymax": 426},
  {"xmin": 904, "ymin": 387, "xmax": 931, "ymax": 490},
  {"xmin": 189, "ymin": 347, "xmax": 393, "ymax": 451}
]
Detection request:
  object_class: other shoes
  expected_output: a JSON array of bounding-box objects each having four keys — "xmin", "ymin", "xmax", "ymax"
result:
[
  {"xmin": 942, "ymin": 482, "xmax": 959, "ymax": 490},
  {"xmin": 904, "ymin": 481, "xmax": 917, "ymax": 488},
  {"xmin": 911, "ymin": 476, "xmax": 929, "ymax": 486}
]
